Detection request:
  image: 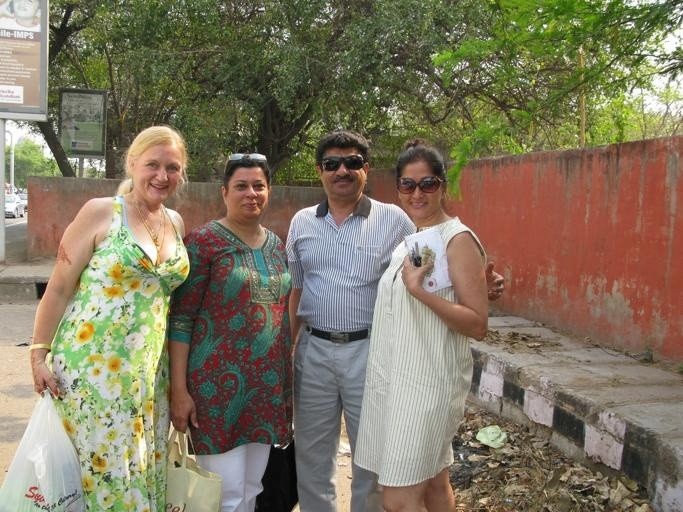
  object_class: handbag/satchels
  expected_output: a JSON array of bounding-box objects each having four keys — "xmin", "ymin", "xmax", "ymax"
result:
[
  {"xmin": 254, "ymin": 441, "xmax": 301, "ymax": 512},
  {"xmin": 403, "ymin": 225, "xmax": 456, "ymax": 296},
  {"xmin": 164, "ymin": 423, "xmax": 224, "ymax": 512}
]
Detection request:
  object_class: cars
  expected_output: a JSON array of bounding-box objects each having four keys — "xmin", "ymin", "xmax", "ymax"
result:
[{"xmin": 4, "ymin": 193, "xmax": 27, "ymax": 218}]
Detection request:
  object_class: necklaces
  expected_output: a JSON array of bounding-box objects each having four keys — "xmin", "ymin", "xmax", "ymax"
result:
[{"xmin": 131, "ymin": 192, "xmax": 168, "ymax": 268}]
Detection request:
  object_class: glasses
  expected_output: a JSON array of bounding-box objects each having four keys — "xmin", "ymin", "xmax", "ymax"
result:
[
  {"xmin": 224, "ymin": 152, "xmax": 269, "ymax": 177},
  {"xmin": 318, "ymin": 153, "xmax": 368, "ymax": 172},
  {"xmin": 396, "ymin": 175, "xmax": 445, "ymax": 195}
]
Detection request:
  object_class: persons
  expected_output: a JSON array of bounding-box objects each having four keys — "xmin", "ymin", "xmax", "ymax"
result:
[
  {"xmin": 26, "ymin": 126, "xmax": 191, "ymax": 511},
  {"xmin": 169, "ymin": 151, "xmax": 293, "ymax": 512},
  {"xmin": 286, "ymin": 130, "xmax": 505, "ymax": 512},
  {"xmin": 352, "ymin": 137, "xmax": 488, "ymax": 511}
]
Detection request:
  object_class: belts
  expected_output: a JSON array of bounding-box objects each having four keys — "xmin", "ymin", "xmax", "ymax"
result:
[{"xmin": 304, "ymin": 324, "xmax": 371, "ymax": 344}]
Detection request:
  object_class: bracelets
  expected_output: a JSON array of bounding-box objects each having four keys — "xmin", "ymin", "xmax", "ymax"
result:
[{"xmin": 28, "ymin": 343, "xmax": 53, "ymax": 353}]
